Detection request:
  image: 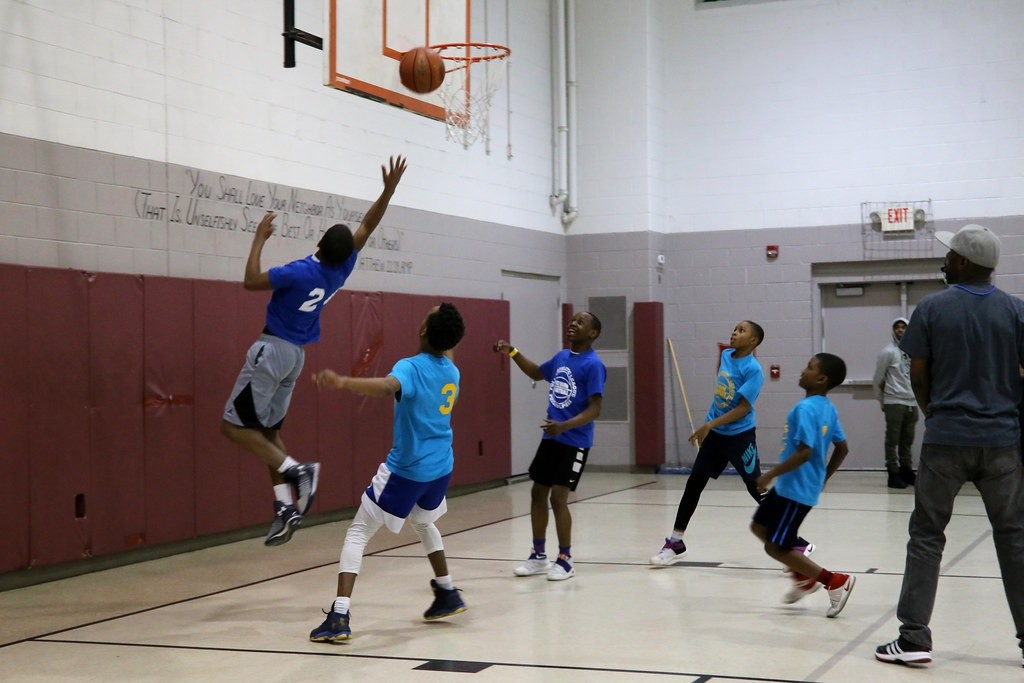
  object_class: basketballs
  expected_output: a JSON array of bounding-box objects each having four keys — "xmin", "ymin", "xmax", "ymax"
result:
[{"xmin": 399, "ymin": 48, "xmax": 445, "ymax": 94}]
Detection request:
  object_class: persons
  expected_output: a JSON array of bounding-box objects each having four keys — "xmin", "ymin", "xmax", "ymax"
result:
[
  {"xmin": 491, "ymin": 311, "xmax": 609, "ymax": 582},
  {"xmin": 309, "ymin": 300, "xmax": 469, "ymax": 641},
  {"xmin": 219, "ymin": 153, "xmax": 410, "ymax": 547},
  {"xmin": 751, "ymin": 351, "xmax": 857, "ymax": 617},
  {"xmin": 873, "ymin": 221, "xmax": 1023, "ymax": 668},
  {"xmin": 874, "ymin": 316, "xmax": 916, "ymax": 490},
  {"xmin": 648, "ymin": 320, "xmax": 817, "ymax": 574}
]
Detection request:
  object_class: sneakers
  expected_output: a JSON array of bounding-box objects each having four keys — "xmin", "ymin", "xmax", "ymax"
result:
[
  {"xmin": 309, "ymin": 602, "xmax": 352, "ymax": 641},
  {"xmin": 783, "ymin": 571, "xmax": 823, "ymax": 603},
  {"xmin": 875, "ymin": 636, "xmax": 932, "ymax": 662},
  {"xmin": 782, "ymin": 535, "xmax": 816, "ymax": 574},
  {"xmin": 424, "ymin": 579, "xmax": 465, "ymax": 621},
  {"xmin": 513, "ymin": 548, "xmax": 548, "ymax": 576},
  {"xmin": 649, "ymin": 538, "xmax": 686, "ymax": 566},
  {"xmin": 292, "ymin": 462, "xmax": 321, "ymax": 515},
  {"xmin": 547, "ymin": 555, "xmax": 574, "ymax": 580},
  {"xmin": 265, "ymin": 500, "xmax": 302, "ymax": 546},
  {"xmin": 826, "ymin": 572, "xmax": 855, "ymax": 618}
]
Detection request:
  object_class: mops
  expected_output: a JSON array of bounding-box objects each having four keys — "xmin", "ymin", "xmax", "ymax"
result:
[{"xmin": 656, "ymin": 338, "xmax": 739, "ymax": 475}]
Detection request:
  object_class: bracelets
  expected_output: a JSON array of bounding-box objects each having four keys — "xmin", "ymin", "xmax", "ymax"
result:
[{"xmin": 509, "ymin": 348, "xmax": 518, "ymax": 357}]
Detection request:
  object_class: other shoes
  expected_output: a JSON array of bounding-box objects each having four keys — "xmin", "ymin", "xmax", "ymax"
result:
[
  {"xmin": 888, "ymin": 472, "xmax": 906, "ymax": 489},
  {"xmin": 901, "ymin": 466, "xmax": 916, "ymax": 487}
]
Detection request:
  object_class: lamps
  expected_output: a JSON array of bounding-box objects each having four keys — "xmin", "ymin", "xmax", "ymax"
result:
[
  {"xmin": 869, "ymin": 206, "xmax": 926, "ymax": 232},
  {"xmin": 835, "ymin": 284, "xmax": 865, "ymax": 297}
]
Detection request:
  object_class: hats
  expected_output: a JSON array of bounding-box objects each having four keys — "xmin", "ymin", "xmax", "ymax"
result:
[{"xmin": 933, "ymin": 225, "xmax": 1001, "ymax": 269}]
